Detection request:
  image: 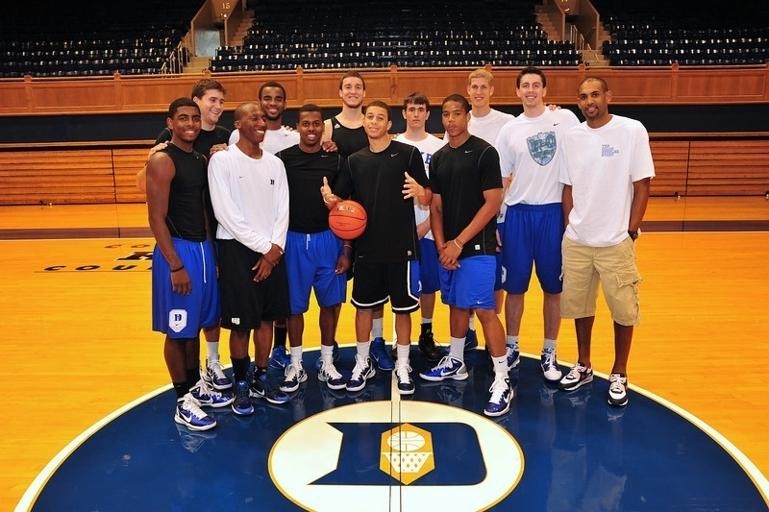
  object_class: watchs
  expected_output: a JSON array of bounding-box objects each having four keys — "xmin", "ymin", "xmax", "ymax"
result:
[{"xmin": 627, "ymin": 229, "xmax": 639, "ymax": 240}]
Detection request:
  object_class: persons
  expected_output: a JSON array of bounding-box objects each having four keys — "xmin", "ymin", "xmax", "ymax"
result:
[
  {"xmin": 320, "ymin": 72, "xmax": 443, "ymax": 395},
  {"xmin": 417, "ymin": 92, "xmax": 516, "ymax": 417},
  {"xmin": 143, "ymin": 78, "xmax": 353, "ymax": 432},
  {"xmin": 494, "ymin": 64, "xmax": 581, "ymax": 382},
  {"xmin": 443, "ymin": 69, "xmax": 515, "ymax": 354},
  {"xmin": 559, "ymin": 74, "xmax": 657, "ymax": 408}
]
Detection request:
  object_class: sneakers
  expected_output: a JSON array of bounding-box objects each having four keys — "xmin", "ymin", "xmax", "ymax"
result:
[
  {"xmin": 268, "ymin": 341, "xmax": 377, "ymax": 392},
  {"xmin": 174, "ymin": 355, "xmax": 289, "ymax": 431},
  {"xmin": 441, "ymin": 328, "xmax": 478, "ymax": 355},
  {"xmin": 483, "ymin": 375, "xmax": 514, "ymax": 417},
  {"xmin": 394, "ymin": 359, "xmax": 415, "ymax": 395},
  {"xmin": 418, "ymin": 332, "xmax": 444, "ymax": 361},
  {"xmin": 369, "ymin": 337, "xmax": 395, "ymax": 371},
  {"xmin": 607, "ymin": 372, "xmax": 628, "ymax": 406},
  {"xmin": 505, "ymin": 343, "xmax": 521, "ymax": 371},
  {"xmin": 541, "ymin": 347, "xmax": 563, "ymax": 385},
  {"xmin": 419, "ymin": 356, "xmax": 469, "ymax": 381},
  {"xmin": 389, "ymin": 345, "xmax": 413, "ymax": 362},
  {"xmin": 558, "ymin": 362, "xmax": 593, "ymax": 391}
]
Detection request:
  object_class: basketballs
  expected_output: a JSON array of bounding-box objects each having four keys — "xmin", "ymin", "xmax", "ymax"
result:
[{"xmin": 328, "ymin": 200, "xmax": 368, "ymax": 240}]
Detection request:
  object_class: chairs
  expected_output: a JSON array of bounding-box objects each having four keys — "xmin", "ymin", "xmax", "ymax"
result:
[
  {"xmin": 601, "ymin": 0, "xmax": 769, "ymax": 65},
  {"xmin": 0, "ymin": 20, "xmax": 190, "ymax": 78}
]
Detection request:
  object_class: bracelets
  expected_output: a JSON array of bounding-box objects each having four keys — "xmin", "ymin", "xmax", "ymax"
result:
[{"xmin": 453, "ymin": 239, "xmax": 465, "ymax": 251}]
